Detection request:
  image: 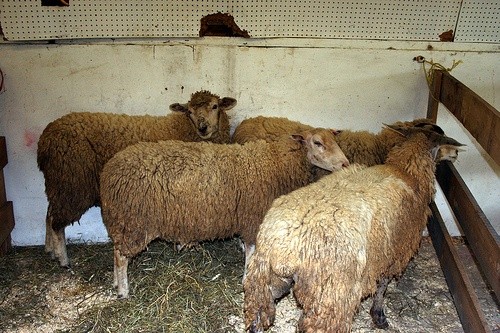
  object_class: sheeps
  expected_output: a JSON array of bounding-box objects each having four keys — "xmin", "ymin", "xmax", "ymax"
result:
[{"xmin": 36, "ymin": 90, "xmax": 470, "ymax": 333}]
